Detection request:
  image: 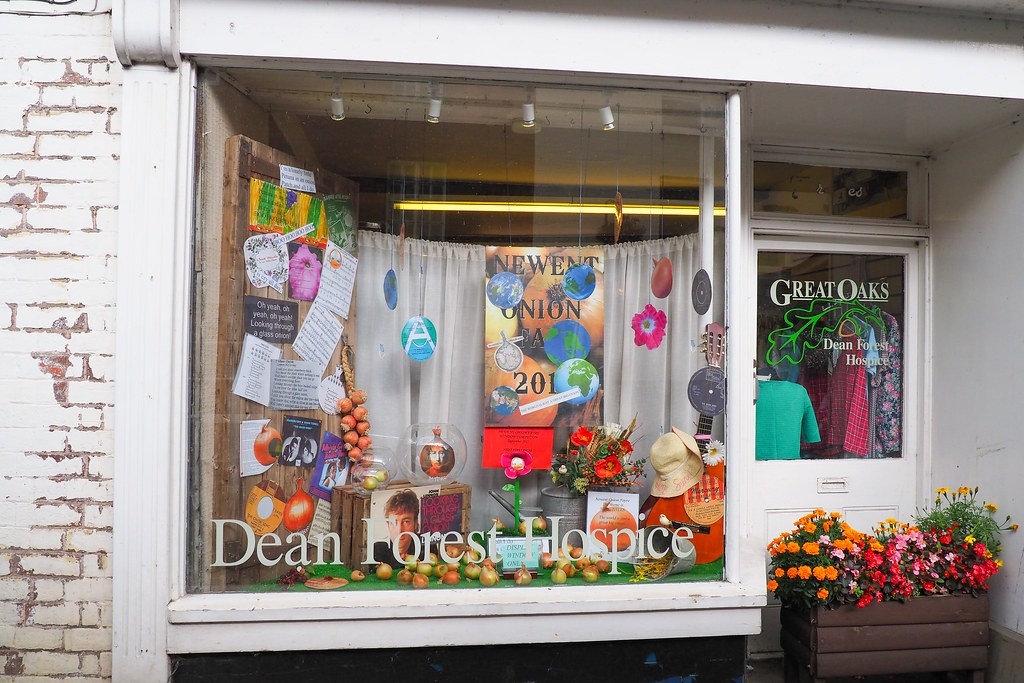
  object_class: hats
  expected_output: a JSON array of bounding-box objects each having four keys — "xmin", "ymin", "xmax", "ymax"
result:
[{"xmin": 650, "ymin": 426, "xmax": 704, "ymax": 498}]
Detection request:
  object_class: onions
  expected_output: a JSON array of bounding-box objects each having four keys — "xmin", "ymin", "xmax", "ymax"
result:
[
  {"xmin": 376, "ymin": 516, "xmax": 610, "ymax": 589},
  {"xmin": 283, "ymin": 478, "xmax": 314, "ymax": 533},
  {"xmin": 651, "ymin": 256, "xmax": 673, "ymax": 299},
  {"xmin": 336, "ymin": 389, "xmax": 374, "ymax": 477},
  {"xmin": 590, "ymin": 501, "xmax": 637, "ymax": 552},
  {"xmin": 254, "ymin": 424, "xmax": 283, "ymax": 466}
]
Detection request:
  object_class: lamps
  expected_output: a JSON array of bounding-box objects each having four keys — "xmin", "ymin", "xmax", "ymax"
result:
[
  {"xmin": 332, "ymin": 86, "xmax": 345, "ymax": 121},
  {"xmin": 600, "ymin": 93, "xmax": 616, "ymax": 130},
  {"xmin": 523, "ymin": 97, "xmax": 535, "ymax": 128},
  {"xmin": 427, "ymin": 96, "xmax": 442, "ymax": 124}
]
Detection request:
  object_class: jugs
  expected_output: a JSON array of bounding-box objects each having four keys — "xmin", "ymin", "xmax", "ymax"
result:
[{"xmin": 488, "ymin": 487, "xmax": 587, "ymax": 548}]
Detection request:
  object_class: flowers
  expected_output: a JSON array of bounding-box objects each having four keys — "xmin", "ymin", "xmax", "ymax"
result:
[
  {"xmin": 703, "ymin": 440, "xmax": 725, "ymax": 467},
  {"xmin": 502, "ymin": 451, "xmax": 533, "ymax": 535},
  {"xmin": 765, "ymin": 485, "xmax": 1017, "ymax": 613},
  {"xmin": 549, "ymin": 415, "xmax": 650, "ymax": 494}
]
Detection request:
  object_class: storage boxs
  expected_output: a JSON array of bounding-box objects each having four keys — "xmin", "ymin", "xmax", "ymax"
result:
[{"xmin": 330, "ymin": 481, "xmax": 471, "ymax": 570}]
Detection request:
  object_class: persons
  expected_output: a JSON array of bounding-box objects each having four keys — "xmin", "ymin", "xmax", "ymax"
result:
[
  {"xmin": 332, "ymin": 458, "xmax": 348, "ymax": 487},
  {"xmin": 322, "ymin": 463, "xmax": 337, "ymax": 487},
  {"xmin": 283, "ymin": 438, "xmax": 298, "ymax": 460},
  {"xmin": 374, "ymin": 492, "xmax": 423, "ymax": 564},
  {"xmin": 756, "ymin": 374, "xmax": 821, "ymax": 458},
  {"xmin": 302, "ymin": 440, "xmax": 315, "ymax": 463},
  {"xmin": 426, "ymin": 443, "xmax": 453, "ymax": 477}
]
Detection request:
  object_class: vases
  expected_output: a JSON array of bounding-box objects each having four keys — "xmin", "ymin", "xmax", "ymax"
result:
[{"xmin": 777, "ymin": 589, "xmax": 992, "ymax": 683}]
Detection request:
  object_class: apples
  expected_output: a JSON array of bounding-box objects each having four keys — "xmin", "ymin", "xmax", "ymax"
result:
[{"xmin": 362, "ymin": 470, "xmax": 388, "ymax": 489}]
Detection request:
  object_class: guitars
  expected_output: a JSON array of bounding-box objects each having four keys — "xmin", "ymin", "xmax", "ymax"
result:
[{"xmin": 635, "ymin": 324, "xmax": 725, "ymax": 564}]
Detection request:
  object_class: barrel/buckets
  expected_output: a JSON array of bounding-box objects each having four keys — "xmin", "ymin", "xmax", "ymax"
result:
[{"xmin": 630, "ymin": 525, "xmax": 698, "ymax": 580}]
